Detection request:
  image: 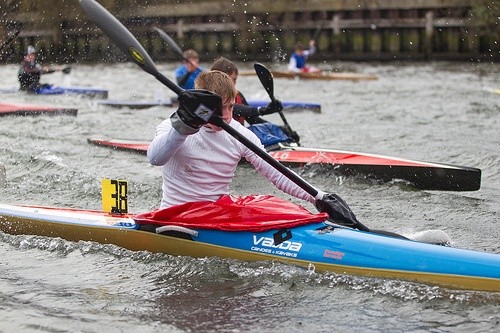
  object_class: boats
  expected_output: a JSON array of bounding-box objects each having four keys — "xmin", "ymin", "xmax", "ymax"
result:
[
  {"xmin": 234, "ymin": 64, "xmax": 380, "ymax": 81},
  {"xmin": 86, "ymin": 132, "xmax": 482, "ymax": 192},
  {"xmin": 0, "ymin": 198, "xmax": 500, "ymax": 293},
  {"xmin": 0, "ymin": 101, "xmax": 79, "ymax": 119},
  {"xmin": 95, "ymin": 97, "xmax": 326, "ymax": 113},
  {"xmin": 0, "ymin": 81, "xmax": 108, "ymax": 98}
]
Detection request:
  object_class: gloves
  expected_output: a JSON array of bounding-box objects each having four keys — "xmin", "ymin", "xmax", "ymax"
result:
[
  {"xmin": 259, "ymin": 99, "xmax": 283, "ymax": 115},
  {"xmin": 170, "ymin": 87, "xmax": 223, "ymax": 134},
  {"xmin": 313, "ymin": 193, "xmax": 359, "ymax": 227},
  {"xmin": 280, "ymin": 126, "xmax": 299, "ymax": 142}
]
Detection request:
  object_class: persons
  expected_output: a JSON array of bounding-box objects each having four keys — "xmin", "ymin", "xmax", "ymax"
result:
[
  {"xmin": 17, "ymin": 44, "xmax": 72, "ymax": 95},
  {"xmin": 210, "ymin": 59, "xmax": 300, "ymax": 148},
  {"xmin": 175, "ymin": 49, "xmax": 208, "ymax": 93},
  {"xmin": 287, "ymin": 39, "xmax": 322, "ymax": 74},
  {"xmin": 146, "ymin": 71, "xmax": 329, "ymax": 211}
]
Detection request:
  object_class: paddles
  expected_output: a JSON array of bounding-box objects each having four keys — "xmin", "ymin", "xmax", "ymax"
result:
[
  {"xmin": 78, "ymin": 0, "xmax": 411, "ymax": 241},
  {"xmin": 0, "ymin": 30, "xmax": 72, "ymax": 74}
]
